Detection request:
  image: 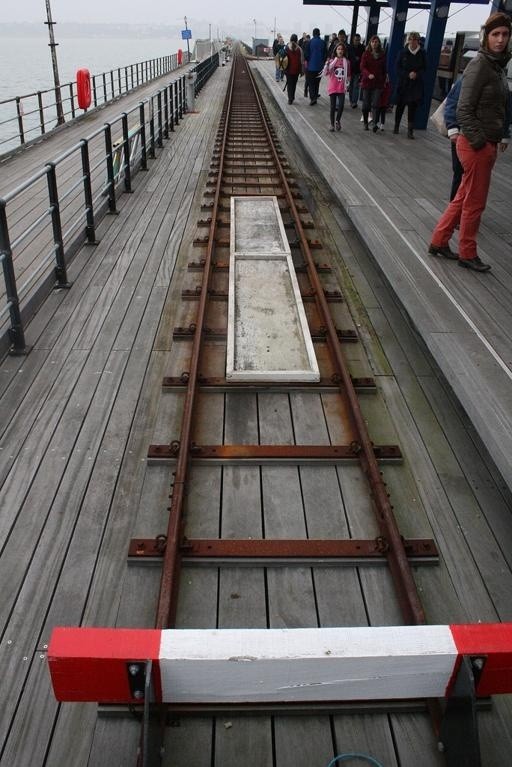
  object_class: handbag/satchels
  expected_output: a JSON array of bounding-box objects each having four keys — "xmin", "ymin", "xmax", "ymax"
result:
[
  {"xmin": 428, "ymin": 97, "xmax": 448, "ymax": 139},
  {"xmin": 276, "ymin": 49, "xmax": 288, "ymax": 70}
]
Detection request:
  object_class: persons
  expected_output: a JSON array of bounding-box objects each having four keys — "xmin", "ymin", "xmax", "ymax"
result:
[
  {"xmin": 441, "ymin": 40, "xmax": 453, "ymax": 54},
  {"xmin": 427, "ymin": 12, "xmax": 511, "ymax": 272},
  {"xmin": 444, "ymin": 74, "xmax": 464, "ymax": 231},
  {"xmin": 273, "ymin": 28, "xmax": 428, "ymax": 140}
]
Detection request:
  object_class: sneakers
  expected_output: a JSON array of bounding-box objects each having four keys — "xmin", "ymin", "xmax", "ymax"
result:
[
  {"xmin": 288, "ymin": 99, "xmax": 292, "ymax": 104},
  {"xmin": 329, "ymin": 125, "xmax": 334, "ymax": 132},
  {"xmin": 276, "ymin": 78, "xmax": 283, "ymax": 81},
  {"xmin": 304, "ymin": 88, "xmax": 320, "ymax": 106},
  {"xmin": 352, "ymin": 104, "xmax": 384, "ymax": 133},
  {"xmin": 336, "ymin": 121, "xmax": 340, "ymax": 130}
]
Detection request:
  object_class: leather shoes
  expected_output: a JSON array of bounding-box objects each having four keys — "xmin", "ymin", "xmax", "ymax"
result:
[
  {"xmin": 394, "ymin": 126, "xmax": 399, "ymax": 134},
  {"xmin": 429, "ymin": 243, "xmax": 460, "ymax": 259},
  {"xmin": 408, "ymin": 129, "xmax": 414, "ymax": 138},
  {"xmin": 458, "ymin": 256, "xmax": 491, "ymax": 272}
]
